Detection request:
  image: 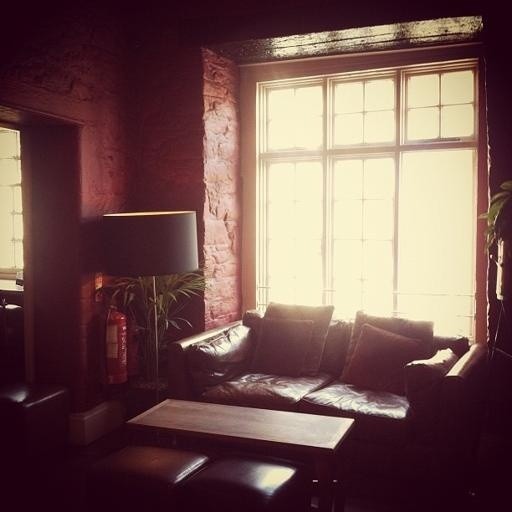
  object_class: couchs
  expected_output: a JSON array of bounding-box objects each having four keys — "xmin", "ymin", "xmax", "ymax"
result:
[{"xmin": 165, "ymin": 300, "xmax": 492, "ymax": 511}]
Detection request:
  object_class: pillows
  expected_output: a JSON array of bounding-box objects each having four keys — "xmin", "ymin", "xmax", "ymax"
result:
[
  {"xmin": 253, "ymin": 301, "xmax": 335, "ymax": 379},
  {"xmin": 339, "ymin": 312, "xmax": 434, "ymax": 394},
  {"xmin": 348, "ymin": 325, "xmax": 422, "ymax": 391},
  {"xmin": 258, "ymin": 320, "xmax": 313, "ymax": 379}
]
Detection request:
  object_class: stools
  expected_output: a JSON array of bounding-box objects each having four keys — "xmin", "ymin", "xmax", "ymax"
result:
[
  {"xmin": 87, "ymin": 444, "xmax": 207, "ymax": 512},
  {"xmin": 179, "ymin": 455, "xmax": 312, "ymax": 512},
  {"xmin": 0, "ymin": 381, "xmax": 70, "ymax": 456}
]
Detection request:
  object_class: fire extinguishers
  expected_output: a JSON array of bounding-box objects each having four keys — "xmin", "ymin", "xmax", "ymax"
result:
[{"xmin": 97, "ymin": 288, "xmax": 130, "ymax": 385}]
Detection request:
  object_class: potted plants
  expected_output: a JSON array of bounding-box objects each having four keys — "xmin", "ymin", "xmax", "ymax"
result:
[{"xmin": 93, "ymin": 269, "xmax": 208, "ymax": 415}]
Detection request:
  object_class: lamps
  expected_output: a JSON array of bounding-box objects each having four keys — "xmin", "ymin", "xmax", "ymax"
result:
[{"xmin": 101, "ymin": 211, "xmax": 200, "ymax": 405}]
